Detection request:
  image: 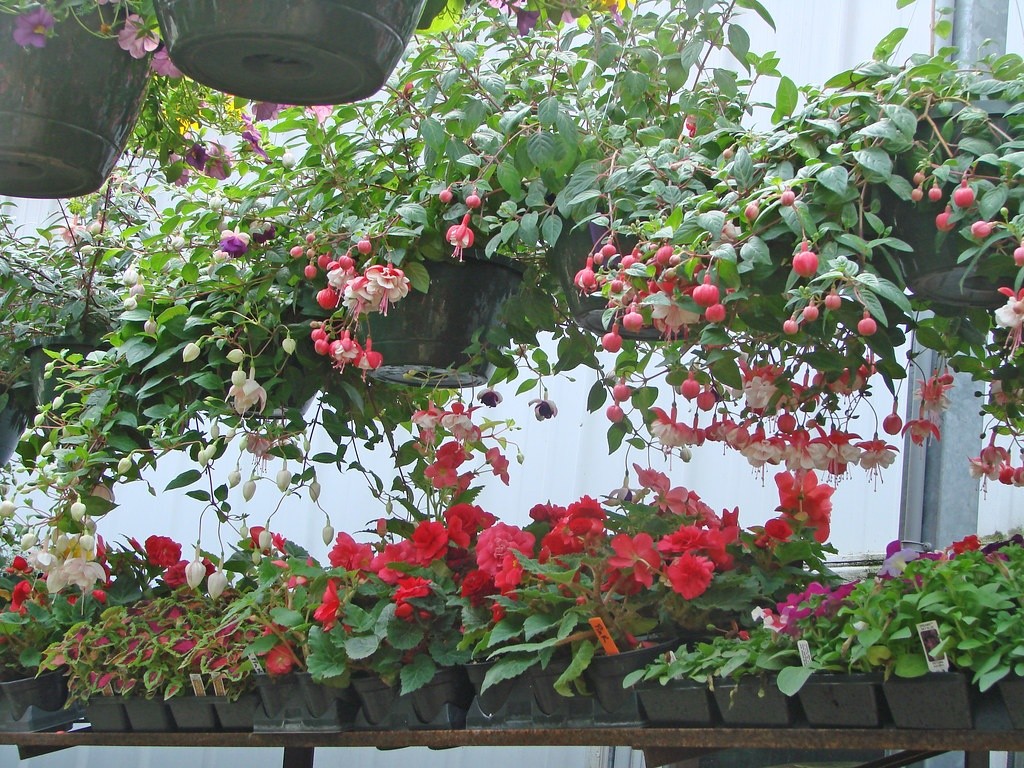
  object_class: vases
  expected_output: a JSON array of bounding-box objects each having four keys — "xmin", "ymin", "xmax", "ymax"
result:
[
  {"xmin": 199, "ymin": 290, "xmax": 324, "ymax": 419},
  {"xmin": 0, "ymin": 632, "xmax": 1024, "ymax": 731},
  {"xmin": 0, "ymin": 385, "xmax": 27, "ymax": 468},
  {"xmin": 24, "ymin": 335, "xmax": 112, "ymax": 435},
  {"xmin": 546, "ymin": 192, "xmax": 684, "ymax": 342},
  {"xmin": 724, "ymin": 233, "xmax": 806, "ymax": 334},
  {"xmin": 1, "ymin": 0, "xmax": 164, "ymax": 199},
  {"xmin": 353, "ymin": 247, "xmax": 527, "ymax": 389},
  {"xmin": 885, "ymin": 98, "xmax": 1024, "ymax": 308},
  {"xmin": 152, "ymin": 1, "xmax": 428, "ymax": 106}
]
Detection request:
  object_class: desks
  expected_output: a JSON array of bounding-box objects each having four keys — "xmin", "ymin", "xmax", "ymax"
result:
[{"xmin": 0, "ymin": 729, "xmax": 1023, "ymax": 768}]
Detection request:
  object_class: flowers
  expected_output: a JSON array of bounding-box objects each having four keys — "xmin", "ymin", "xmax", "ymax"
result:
[{"xmin": 0, "ymin": 0, "xmax": 1024, "ymax": 710}]
